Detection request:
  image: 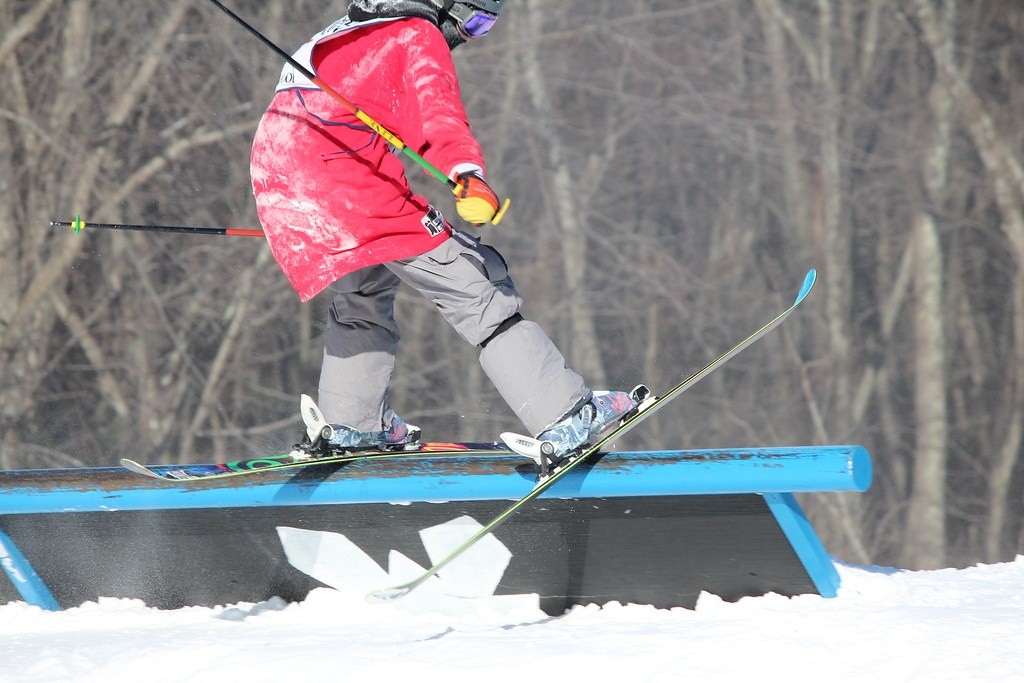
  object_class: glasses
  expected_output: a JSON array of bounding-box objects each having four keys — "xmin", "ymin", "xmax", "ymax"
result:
[{"xmin": 462, "ymin": 13, "xmax": 497, "ymax": 38}]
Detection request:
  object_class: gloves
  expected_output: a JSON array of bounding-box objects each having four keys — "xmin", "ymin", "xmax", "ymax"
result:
[{"xmin": 452, "ymin": 172, "xmax": 500, "ymax": 226}]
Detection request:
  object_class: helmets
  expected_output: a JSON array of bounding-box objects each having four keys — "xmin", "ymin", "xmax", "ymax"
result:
[{"xmin": 427, "ymin": 0, "xmax": 503, "ymax": 26}]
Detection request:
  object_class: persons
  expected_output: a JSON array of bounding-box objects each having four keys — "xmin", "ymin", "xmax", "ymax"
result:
[{"xmin": 249, "ymin": 0, "xmax": 640, "ymax": 467}]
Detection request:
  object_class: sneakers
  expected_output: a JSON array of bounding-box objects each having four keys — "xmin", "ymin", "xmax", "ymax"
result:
[
  {"xmin": 538, "ymin": 390, "xmax": 636, "ymax": 461},
  {"xmin": 324, "ymin": 415, "xmax": 422, "ymax": 449}
]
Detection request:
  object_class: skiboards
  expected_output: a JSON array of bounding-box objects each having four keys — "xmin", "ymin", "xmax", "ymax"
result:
[{"xmin": 118, "ymin": 267, "xmax": 818, "ymax": 604}]
